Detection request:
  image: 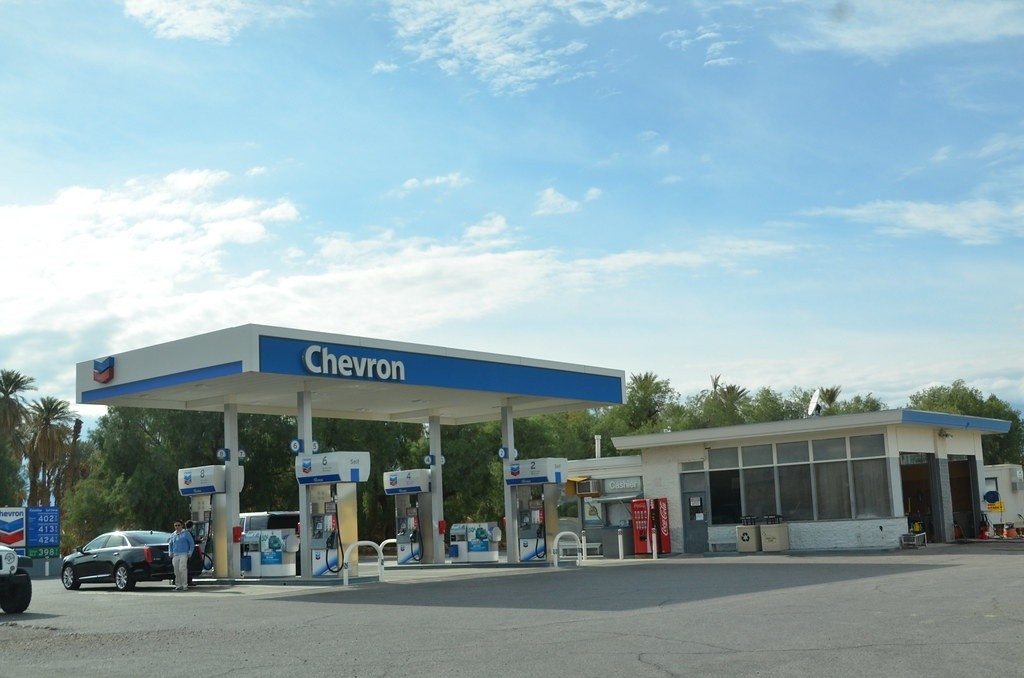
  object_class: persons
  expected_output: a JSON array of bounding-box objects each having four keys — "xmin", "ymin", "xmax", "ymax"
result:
[
  {"xmin": 185, "ymin": 520, "xmax": 208, "ymax": 586},
  {"xmin": 169, "ymin": 520, "xmax": 194, "ymax": 590}
]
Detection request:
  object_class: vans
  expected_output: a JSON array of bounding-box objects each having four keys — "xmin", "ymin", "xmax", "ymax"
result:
[{"xmin": 197, "ymin": 510, "xmax": 300, "ymax": 560}]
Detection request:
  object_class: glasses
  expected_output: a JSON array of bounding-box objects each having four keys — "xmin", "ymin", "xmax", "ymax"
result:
[{"xmin": 174, "ymin": 524, "xmax": 182, "ymax": 527}]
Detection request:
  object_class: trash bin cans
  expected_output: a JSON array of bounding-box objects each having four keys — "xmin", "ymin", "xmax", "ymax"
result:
[
  {"xmin": 760, "ymin": 514, "xmax": 790, "ymax": 552},
  {"xmin": 736, "ymin": 515, "xmax": 761, "ymax": 552}
]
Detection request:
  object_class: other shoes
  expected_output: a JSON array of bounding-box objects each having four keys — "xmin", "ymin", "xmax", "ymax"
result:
[
  {"xmin": 189, "ymin": 581, "xmax": 196, "ymax": 585},
  {"xmin": 183, "ymin": 587, "xmax": 189, "ymax": 591},
  {"xmin": 173, "ymin": 587, "xmax": 182, "ymax": 591}
]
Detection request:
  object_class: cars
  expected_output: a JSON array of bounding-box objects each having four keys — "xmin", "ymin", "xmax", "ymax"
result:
[{"xmin": 60, "ymin": 529, "xmax": 204, "ymax": 592}]
